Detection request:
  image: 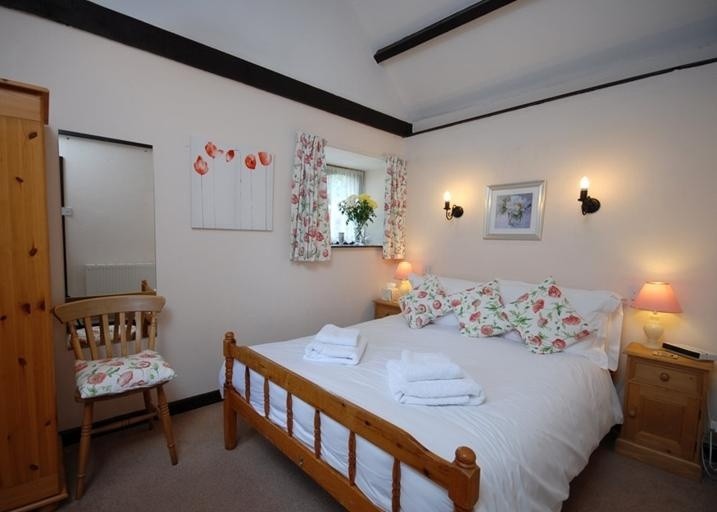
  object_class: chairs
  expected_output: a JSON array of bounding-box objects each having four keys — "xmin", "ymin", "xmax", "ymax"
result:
[
  {"xmin": 54, "ymin": 295, "xmax": 177, "ymax": 499},
  {"xmin": 68, "ymin": 291, "xmax": 156, "ymax": 345}
]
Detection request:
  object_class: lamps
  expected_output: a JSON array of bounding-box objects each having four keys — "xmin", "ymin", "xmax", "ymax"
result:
[
  {"xmin": 443, "ymin": 191, "xmax": 463, "ymax": 220},
  {"xmin": 577, "ymin": 176, "xmax": 600, "ymax": 215},
  {"xmin": 393, "ymin": 262, "xmax": 413, "ymax": 296},
  {"xmin": 630, "ymin": 281, "xmax": 682, "ymax": 350}
]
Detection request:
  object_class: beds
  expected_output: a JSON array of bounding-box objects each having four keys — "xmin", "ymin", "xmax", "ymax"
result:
[{"xmin": 218, "ymin": 272, "xmax": 623, "ymax": 512}]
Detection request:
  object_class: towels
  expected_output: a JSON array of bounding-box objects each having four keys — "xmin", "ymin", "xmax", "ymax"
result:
[
  {"xmin": 386, "ymin": 350, "xmax": 486, "ymax": 406},
  {"xmin": 303, "ymin": 324, "xmax": 366, "ymax": 365}
]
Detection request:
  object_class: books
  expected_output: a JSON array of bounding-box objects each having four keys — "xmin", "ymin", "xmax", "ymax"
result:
[{"xmin": 661, "ymin": 341, "xmax": 717, "ymax": 361}]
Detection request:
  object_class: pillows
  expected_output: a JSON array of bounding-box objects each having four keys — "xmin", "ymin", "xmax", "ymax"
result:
[
  {"xmin": 398, "ymin": 275, "xmax": 623, "ymax": 372},
  {"xmin": 73, "ymin": 349, "xmax": 177, "ymax": 399},
  {"xmin": 68, "ymin": 325, "xmax": 137, "ymax": 347}
]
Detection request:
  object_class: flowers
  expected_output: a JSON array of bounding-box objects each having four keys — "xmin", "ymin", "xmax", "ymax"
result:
[{"xmin": 338, "ymin": 193, "xmax": 378, "ymax": 238}]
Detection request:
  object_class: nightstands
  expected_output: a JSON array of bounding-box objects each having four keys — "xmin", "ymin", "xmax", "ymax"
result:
[
  {"xmin": 614, "ymin": 342, "xmax": 713, "ymax": 482},
  {"xmin": 372, "ymin": 300, "xmax": 402, "ymax": 319}
]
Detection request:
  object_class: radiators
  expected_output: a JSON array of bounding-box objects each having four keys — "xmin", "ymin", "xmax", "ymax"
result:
[{"xmin": 85, "ymin": 262, "xmax": 156, "ymax": 321}]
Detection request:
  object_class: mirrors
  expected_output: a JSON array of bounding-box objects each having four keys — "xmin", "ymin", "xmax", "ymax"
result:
[{"xmin": 58, "ymin": 134, "xmax": 156, "ymax": 326}]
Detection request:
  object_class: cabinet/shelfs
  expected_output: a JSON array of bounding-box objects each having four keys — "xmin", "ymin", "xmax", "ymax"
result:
[{"xmin": 0, "ymin": 77, "xmax": 68, "ymax": 512}]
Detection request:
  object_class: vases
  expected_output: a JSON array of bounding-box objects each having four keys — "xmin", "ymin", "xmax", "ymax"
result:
[{"xmin": 354, "ymin": 227, "xmax": 365, "ymax": 245}]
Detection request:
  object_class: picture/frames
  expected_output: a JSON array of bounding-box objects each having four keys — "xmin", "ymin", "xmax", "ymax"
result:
[{"xmin": 482, "ymin": 179, "xmax": 546, "ymax": 241}]
